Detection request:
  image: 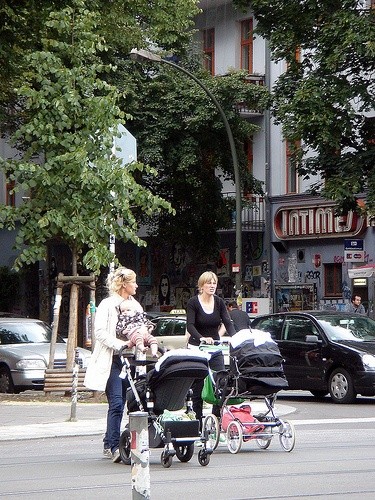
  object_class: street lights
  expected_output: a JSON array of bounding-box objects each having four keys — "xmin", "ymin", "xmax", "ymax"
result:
[{"xmin": 128, "ymin": 47, "xmax": 243, "ymax": 312}]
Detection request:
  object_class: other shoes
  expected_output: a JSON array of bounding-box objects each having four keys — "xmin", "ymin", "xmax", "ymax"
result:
[
  {"xmin": 103, "ymin": 449, "xmax": 113, "ymax": 459},
  {"xmin": 112, "ymin": 449, "xmax": 121, "ymax": 463}
]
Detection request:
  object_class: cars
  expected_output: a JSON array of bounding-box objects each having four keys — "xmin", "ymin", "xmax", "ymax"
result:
[
  {"xmin": 250, "ymin": 311, "xmax": 375, "ymax": 403},
  {"xmin": 144, "ymin": 311, "xmax": 170, "ymax": 319},
  {"xmin": 0, "ymin": 317, "xmax": 92, "ymax": 394},
  {"xmin": 145, "ymin": 315, "xmax": 190, "ymax": 356}
]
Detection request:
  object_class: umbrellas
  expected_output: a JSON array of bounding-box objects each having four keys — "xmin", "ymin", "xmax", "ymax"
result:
[{"xmin": 347, "ymin": 264, "xmax": 375, "ymax": 279}]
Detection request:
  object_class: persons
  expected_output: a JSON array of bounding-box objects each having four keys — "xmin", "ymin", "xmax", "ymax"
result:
[
  {"xmin": 116, "ymin": 299, "xmax": 158, "ymax": 358},
  {"xmin": 345, "ymin": 295, "xmax": 365, "ymax": 315},
  {"xmin": 83, "ymin": 266, "xmax": 152, "ymax": 463},
  {"xmin": 219, "ymin": 301, "xmax": 251, "ymax": 336},
  {"xmin": 186, "ymin": 272, "xmax": 238, "ymax": 447}
]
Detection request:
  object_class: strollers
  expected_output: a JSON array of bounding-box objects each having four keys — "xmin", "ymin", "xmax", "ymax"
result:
[
  {"xmin": 119, "ymin": 341, "xmax": 214, "ymax": 468},
  {"xmin": 199, "ymin": 337, "xmax": 296, "ymax": 455}
]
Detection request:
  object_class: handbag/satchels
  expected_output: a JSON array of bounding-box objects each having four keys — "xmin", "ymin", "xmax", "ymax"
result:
[
  {"xmin": 201, "ymin": 372, "xmax": 245, "ymax": 405},
  {"xmin": 222, "ymin": 405, "xmax": 265, "ymax": 435},
  {"xmin": 156, "ymin": 409, "xmax": 191, "ymax": 431}
]
